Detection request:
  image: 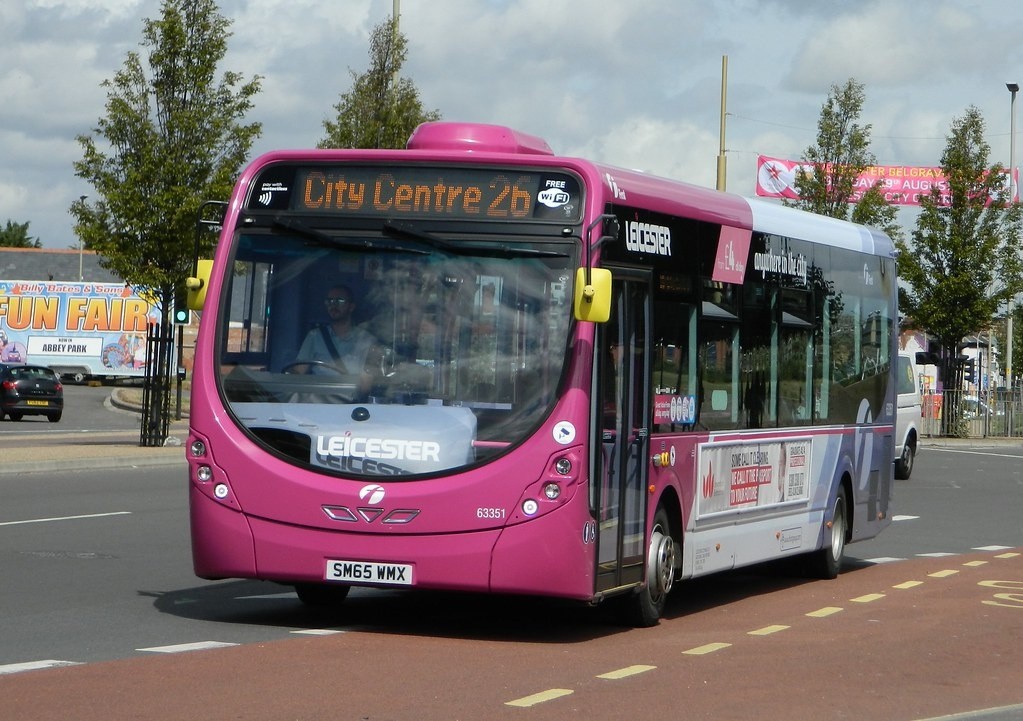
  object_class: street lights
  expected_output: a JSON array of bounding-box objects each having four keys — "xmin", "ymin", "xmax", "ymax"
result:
[
  {"xmin": 77, "ymin": 194, "xmax": 90, "ymax": 283},
  {"xmin": 1006, "ymin": 82, "xmax": 1021, "ymax": 397}
]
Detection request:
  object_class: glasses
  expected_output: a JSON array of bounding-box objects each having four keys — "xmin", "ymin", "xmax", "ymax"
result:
[{"xmin": 324, "ymin": 297, "xmax": 351, "ymax": 305}]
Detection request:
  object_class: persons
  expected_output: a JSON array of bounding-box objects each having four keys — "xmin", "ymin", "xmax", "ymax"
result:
[{"xmin": 289, "ymin": 286, "xmax": 389, "ymax": 395}]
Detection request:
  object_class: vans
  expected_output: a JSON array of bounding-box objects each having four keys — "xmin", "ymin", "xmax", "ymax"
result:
[{"xmin": 895, "ymin": 352, "xmax": 924, "ymax": 479}]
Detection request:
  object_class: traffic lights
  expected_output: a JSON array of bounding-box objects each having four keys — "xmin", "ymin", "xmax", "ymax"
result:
[
  {"xmin": 915, "ymin": 340, "xmax": 941, "ymax": 367},
  {"xmin": 963, "ymin": 358, "xmax": 974, "ymax": 385},
  {"xmin": 171, "ymin": 282, "xmax": 192, "ymax": 326}
]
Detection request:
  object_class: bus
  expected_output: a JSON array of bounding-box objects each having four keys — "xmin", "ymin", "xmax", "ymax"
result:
[{"xmin": 186, "ymin": 121, "xmax": 901, "ymax": 628}]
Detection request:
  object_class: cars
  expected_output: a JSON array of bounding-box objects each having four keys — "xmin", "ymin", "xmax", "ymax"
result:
[{"xmin": 0, "ymin": 359, "xmax": 64, "ymax": 423}]
